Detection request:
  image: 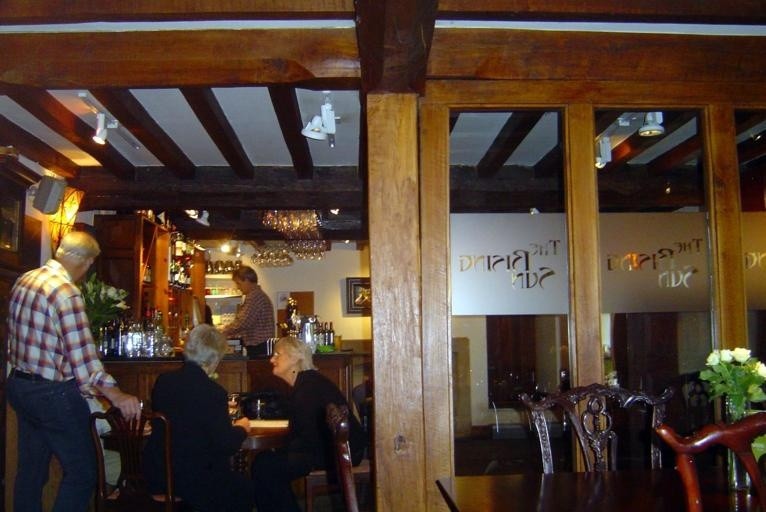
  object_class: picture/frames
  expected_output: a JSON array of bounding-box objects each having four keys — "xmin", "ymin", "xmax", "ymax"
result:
[{"xmin": 346, "ymin": 276, "xmax": 373, "ymax": 314}]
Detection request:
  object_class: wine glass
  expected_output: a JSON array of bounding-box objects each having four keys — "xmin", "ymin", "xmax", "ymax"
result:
[{"xmin": 250, "ymin": 211, "xmax": 328, "ymax": 267}]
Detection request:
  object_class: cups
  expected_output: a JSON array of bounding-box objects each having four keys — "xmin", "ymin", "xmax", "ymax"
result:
[
  {"xmin": 204, "ymin": 287, "xmax": 240, "ymax": 295},
  {"xmin": 253, "ymin": 399, "xmax": 265, "ymax": 419},
  {"xmin": 228, "ymin": 393, "xmax": 241, "ymax": 417}
]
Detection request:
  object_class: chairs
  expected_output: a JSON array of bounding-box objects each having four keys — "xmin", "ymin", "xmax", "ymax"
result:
[
  {"xmin": 95, "ymin": 402, "xmax": 186, "ymax": 509},
  {"xmin": 295, "ymin": 378, "xmax": 378, "ymax": 512},
  {"xmin": 652, "ymin": 412, "xmax": 766, "ymax": 511},
  {"xmin": 517, "ymin": 386, "xmax": 674, "ymax": 473},
  {"xmin": 638, "ymin": 372, "xmax": 723, "ymax": 467}
]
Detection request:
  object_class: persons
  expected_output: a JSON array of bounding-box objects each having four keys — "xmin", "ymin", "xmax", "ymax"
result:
[
  {"xmin": 249, "ymin": 332, "xmax": 365, "ymax": 510},
  {"xmin": 138, "ymin": 322, "xmax": 256, "ymax": 511},
  {"xmin": 3, "ymin": 228, "xmax": 144, "ymax": 512},
  {"xmin": 216, "ymin": 264, "xmax": 277, "ymax": 356}
]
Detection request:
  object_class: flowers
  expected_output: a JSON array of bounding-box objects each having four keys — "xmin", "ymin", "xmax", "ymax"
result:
[
  {"xmin": 77, "ymin": 272, "xmax": 131, "ymax": 335},
  {"xmin": 701, "ymin": 344, "xmax": 766, "ymax": 484}
]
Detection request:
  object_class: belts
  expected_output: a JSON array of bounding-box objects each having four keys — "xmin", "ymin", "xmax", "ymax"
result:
[{"xmin": 10, "ymin": 368, "xmax": 47, "ymax": 383}]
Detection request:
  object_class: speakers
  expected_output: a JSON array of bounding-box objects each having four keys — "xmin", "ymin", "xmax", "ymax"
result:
[{"xmin": 32, "ymin": 176, "xmax": 67, "ymax": 215}]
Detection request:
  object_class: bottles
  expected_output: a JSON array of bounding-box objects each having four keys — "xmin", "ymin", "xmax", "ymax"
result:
[
  {"xmin": 205, "ymin": 259, "xmax": 242, "ymax": 274},
  {"xmin": 139, "ymin": 256, "xmax": 190, "ymax": 291},
  {"xmin": 279, "ymin": 296, "xmax": 335, "ymax": 345},
  {"xmin": 100, "ymin": 300, "xmax": 187, "ymax": 358},
  {"xmin": 211, "ymin": 303, "xmax": 234, "ymax": 326},
  {"xmin": 139, "ymin": 399, "xmax": 144, "ymax": 415}
]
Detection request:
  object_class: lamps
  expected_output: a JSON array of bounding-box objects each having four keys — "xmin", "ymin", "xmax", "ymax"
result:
[
  {"xmin": 184, "ymin": 206, "xmax": 212, "ymax": 227},
  {"xmin": 49, "ymin": 185, "xmax": 84, "ymax": 256},
  {"xmin": 594, "ymin": 111, "xmax": 663, "ymax": 170},
  {"xmin": 301, "ymin": 102, "xmax": 338, "ymax": 142},
  {"xmin": 92, "ymin": 109, "xmax": 110, "ymax": 145}
]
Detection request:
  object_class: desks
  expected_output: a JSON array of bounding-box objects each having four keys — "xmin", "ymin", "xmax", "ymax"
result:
[{"xmin": 433, "ymin": 471, "xmax": 766, "ymax": 511}]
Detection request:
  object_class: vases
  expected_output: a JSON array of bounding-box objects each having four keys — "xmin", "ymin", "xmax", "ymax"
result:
[{"xmin": 725, "ymin": 393, "xmax": 752, "ymax": 490}]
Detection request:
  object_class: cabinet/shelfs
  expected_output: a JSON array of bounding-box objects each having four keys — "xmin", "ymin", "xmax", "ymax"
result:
[
  {"xmin": 203, "ymin": 274, "xmax": 242, "ymax": 301},
  {"xmin": 93, "ymin": 212, "xmax": 205, "ymax": 338}
]
